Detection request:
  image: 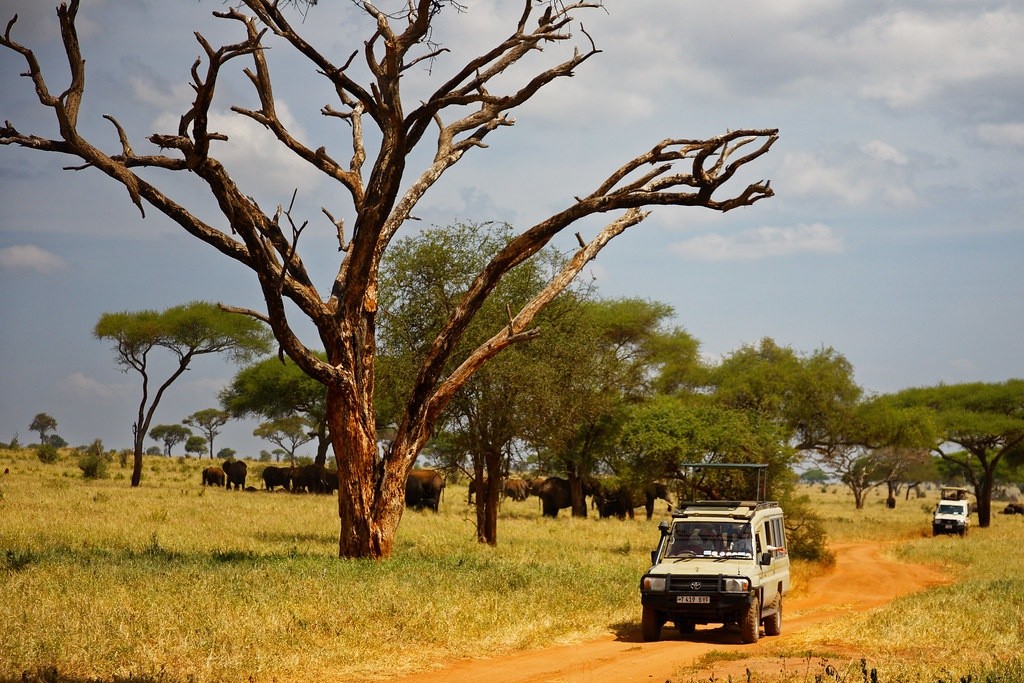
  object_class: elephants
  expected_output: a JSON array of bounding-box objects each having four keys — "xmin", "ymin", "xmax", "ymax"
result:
[
  {"xmin": 469, "ymin": 476, "xmax": 675, "ymax": 522},
  {"xmin": 200, "ymin": 461, "xmax": 247, "ymax": 491},
  {"xmin": 261, "ymin": 466, "xmax": 447, "ymax": 513}
]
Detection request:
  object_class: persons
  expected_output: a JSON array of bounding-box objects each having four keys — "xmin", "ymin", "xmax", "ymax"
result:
[
  {"xmin": 730, "ymin": 525, "xmax": 752, "ymax": 552},
  {"xmin": 688, "ymin": 534, "xmax": 703, "ymax": 549}
]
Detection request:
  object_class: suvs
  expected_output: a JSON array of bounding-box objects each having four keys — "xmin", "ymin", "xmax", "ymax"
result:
[
  {"xmin": 636, "ymin": 500, "xmax": 792, "ymax": 645},
  {"xmin": 930, "ymin": 498, "xmax": 970, "ymax": 538}
]
